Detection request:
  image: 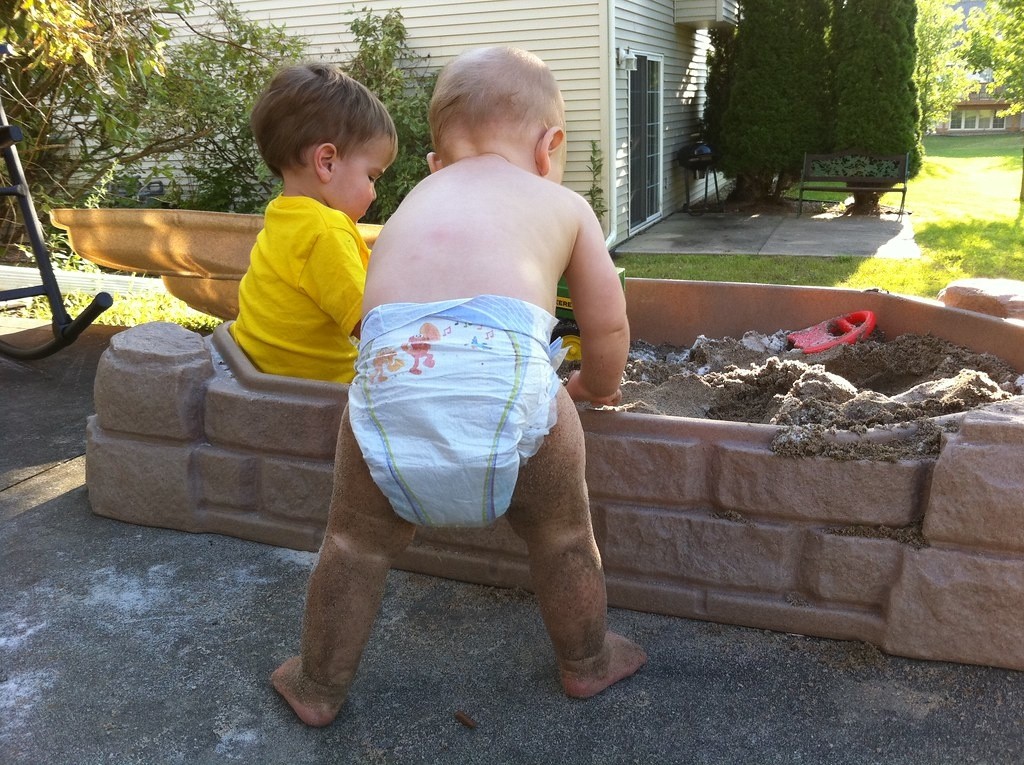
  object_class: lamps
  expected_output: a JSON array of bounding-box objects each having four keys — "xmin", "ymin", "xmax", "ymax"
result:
[{"xmin": 618, "ymin": 45, "xmax": 639, "ymax": 71}]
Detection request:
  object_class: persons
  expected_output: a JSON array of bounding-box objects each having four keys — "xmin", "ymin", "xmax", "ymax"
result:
[
  {"xmin": 229, "ymin": 65, "xmax": 399, "ymax": 382},
  {"xmin": 270, "ymin": 45, "xmax": 646, "ymax": 727}
]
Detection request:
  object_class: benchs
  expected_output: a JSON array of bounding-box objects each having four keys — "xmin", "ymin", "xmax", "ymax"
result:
[{"xmin": 797, "ymin": 147, "xmax": 911, "ymax": 222}]
